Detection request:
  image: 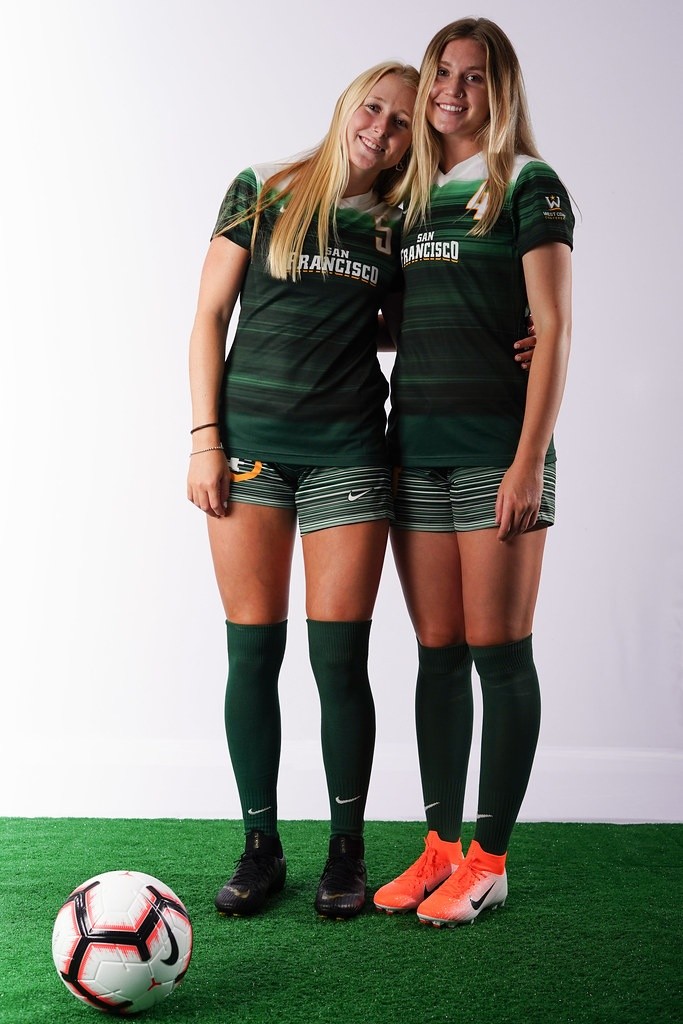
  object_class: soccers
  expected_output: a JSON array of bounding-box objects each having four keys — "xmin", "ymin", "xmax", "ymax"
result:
[{"xmin": 51, "ymin": 870, "xmax": 194, "ymax": 1015}]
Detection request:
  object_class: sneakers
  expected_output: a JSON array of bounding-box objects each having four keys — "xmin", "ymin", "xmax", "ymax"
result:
[
  {"xmin": 213, "ymin": 831, "xmax": 286, "ymax": 918},
  {"xmin": 416, "ymin": 866, "xmax": 509, "ymax": 929},
  {"xmin": 314, "ymin": 833, "xmax": 368, "ymax": 923},
  {"xmin": 372, "ymin": 851, "xmax": 466, "ymax": 916}
]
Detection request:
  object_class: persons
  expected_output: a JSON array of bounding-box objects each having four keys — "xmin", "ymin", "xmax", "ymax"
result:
[
  {"xmin": 186, "ymin": 62, "xmax": 537, "ymax": 920},
  {"xmin": 375, "ymin": 14, "xmax": 579, "ymax": 929}
]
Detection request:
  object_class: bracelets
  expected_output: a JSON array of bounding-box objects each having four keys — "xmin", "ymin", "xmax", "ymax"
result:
[
  {"xmin": 189, "ymin": 447, "xmax": 224, "ymax": 457},
  {"xmin": 191, "ymin": 423, "xmax": 221, "ymax": 434}
]
